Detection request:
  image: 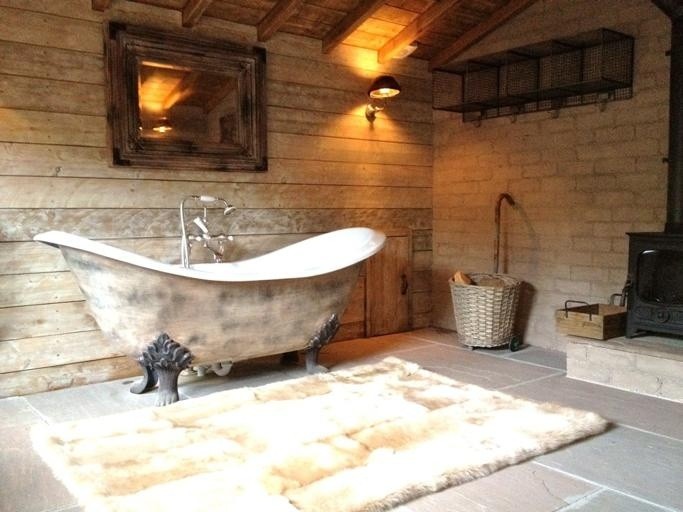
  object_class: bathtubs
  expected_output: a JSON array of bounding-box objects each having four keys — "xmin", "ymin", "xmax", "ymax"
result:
[{"xmin": 33, "ymin": 226, "xmax": 387, "ymax": 408}]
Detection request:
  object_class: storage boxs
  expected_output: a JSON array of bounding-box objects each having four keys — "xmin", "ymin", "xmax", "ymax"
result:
[{"xmin": 555, "ymin": 292, "xmax": 627, "ymax": 341}]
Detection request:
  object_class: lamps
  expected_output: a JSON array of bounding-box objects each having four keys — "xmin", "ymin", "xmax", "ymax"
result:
[
  {"xmin": 363, "ymin": 74, "xmax": 400, "ymax": 121},
  {"xmin": 152, "ymin": 118, "xmax": 173, "ymax": 133}
]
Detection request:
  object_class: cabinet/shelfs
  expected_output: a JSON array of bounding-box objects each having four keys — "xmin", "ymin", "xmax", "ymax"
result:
[{"xmin": 429, "ymin": 28, "xmax": 634, "ymax": 112}]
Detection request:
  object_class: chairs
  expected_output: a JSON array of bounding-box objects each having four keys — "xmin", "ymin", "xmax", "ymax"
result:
[{"xmin": 102, "ymin": 21, "xmax": 266, "ymax": 173}]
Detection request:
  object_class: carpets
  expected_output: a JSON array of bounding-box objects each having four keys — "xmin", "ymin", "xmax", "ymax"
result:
[{"xmin": 35, "ymin": 356, "xmax": 608, "ymax": 510}]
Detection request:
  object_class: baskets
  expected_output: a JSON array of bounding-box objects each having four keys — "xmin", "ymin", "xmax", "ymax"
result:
[{"xmin": 449, "ymin": 273, "xmax": 522, "ymax": 348}]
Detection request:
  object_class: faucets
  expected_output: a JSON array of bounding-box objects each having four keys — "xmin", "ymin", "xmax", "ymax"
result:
[{"xmin": 187, "ymin": 233, "xmax": 234, "ymax": 262}]
